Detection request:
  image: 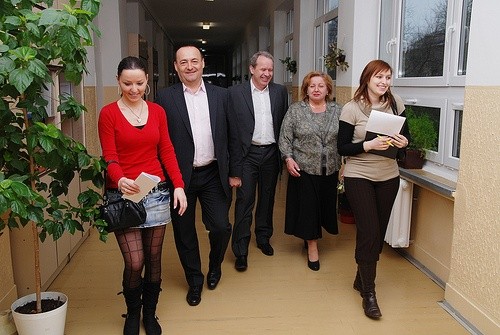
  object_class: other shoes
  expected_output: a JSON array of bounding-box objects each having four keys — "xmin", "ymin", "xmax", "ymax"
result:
[
  {"xmin": 363, "ymin": 292, "xmax": 381, "ymax": 318},
  {"xmin": 353, "ymin": 280, "xmax": 361, "ymax": 292}
]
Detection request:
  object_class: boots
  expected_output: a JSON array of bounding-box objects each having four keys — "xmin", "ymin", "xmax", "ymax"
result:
[
  {"xmin": 117, "ymin": 283, "xmax": 145, "ymax": 335},
  {"xmin": 143, "ymin": 279, "xmax": 162, "ymax": 335}
]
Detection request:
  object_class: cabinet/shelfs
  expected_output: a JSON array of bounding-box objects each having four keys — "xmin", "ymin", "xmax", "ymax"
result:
[{"xmin": 8, "ymin": 59, "xmax": 90, "ymax": 300}]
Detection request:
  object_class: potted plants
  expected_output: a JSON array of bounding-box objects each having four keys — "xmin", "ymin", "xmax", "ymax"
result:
[
  {"xmin": 399, "ymin": 107, "xmax": 437, "ymax": 169},
  {"xmin": 0, "ymin": 0, "xmax": 109, "ymax": 335},
  {"xmin": 277, "ymin": 56, "xmax": 297, "ymax": 74},
  {"xmin": 322, "ymin": 42, "xmax": 349, "ymax": 72}
]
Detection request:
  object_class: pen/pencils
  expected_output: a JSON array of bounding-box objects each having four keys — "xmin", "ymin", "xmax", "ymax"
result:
[{"xmin": 377, "ymin": 135, "xmax": 394, "ymax": 147}]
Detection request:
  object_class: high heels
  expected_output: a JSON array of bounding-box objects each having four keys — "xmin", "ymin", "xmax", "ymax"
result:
[{"xmin": 308, "ymin": 258, "xmax": 320, "ymax": 271}]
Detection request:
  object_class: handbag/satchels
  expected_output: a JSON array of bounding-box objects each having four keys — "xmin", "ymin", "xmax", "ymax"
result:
[{"xmin": 99, "ymin": 160, "xmax": 146, "ymax": 233}]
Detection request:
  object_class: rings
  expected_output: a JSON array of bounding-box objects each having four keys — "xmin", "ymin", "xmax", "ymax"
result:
[{"xmin": 125, "ymin": 189, "xmax": 128, "ymax": 192}]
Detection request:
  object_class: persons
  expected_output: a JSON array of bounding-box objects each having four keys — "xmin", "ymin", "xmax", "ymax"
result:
[
  {"xmin": 278, "ymin": 71, "xmax": 343, "ymax": 271},
  {"xmin": 230, "ymin": 51, "xmax": 288, "ymax": 272},
  {"xmin": 337, "ymin": 60, "xmax": 410, "ymax": 318},
  {"xmin": 98, "ymin": 56, "xmax": 187, "ymax": 335},
  {"xmin": 154, "ymin": 43, "xmax": 231, "ymax": 306}
]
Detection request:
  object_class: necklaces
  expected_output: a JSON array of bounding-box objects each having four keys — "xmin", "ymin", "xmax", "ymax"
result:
[
  {"xmin": 122, "ymin": 101, "xmax": 143, "ymax": 122},
  {"xmin": 310, "ymin": 103, "xmax": 326, "ymax": 109}
]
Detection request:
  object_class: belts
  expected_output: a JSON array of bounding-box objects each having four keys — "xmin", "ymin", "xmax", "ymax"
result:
[
  {"xmin": 193, "ymin": 163, "xmax": 212, "ymax": 171},
  {"xmin": 251, "ymin": 143, "xmax": 274, "ymax": 148},
  {"xmin": 104, "ymin": 184, "xmax": 170, "ymax": 194}
]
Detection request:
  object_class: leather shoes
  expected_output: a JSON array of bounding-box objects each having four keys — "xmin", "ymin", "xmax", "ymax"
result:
[
  {"xmin": 186, "ymin": 285, "xmax": 202, "ymax": 306},
  {"xmin": 259, "ymin": 243, "xmax": 273, "ymax": 255},
  {"xmin": 207, "ymin": 272, "xmax": 221, "ymax": 290},
  {"xmin": 235, "ymin": 255, "xmax": 247, "ymax": 271}
]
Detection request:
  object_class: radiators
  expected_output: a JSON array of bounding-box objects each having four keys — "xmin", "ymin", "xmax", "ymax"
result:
[{"xmin": 383, "ymin": 178, "xmax": 413, "ymax": 248}]
